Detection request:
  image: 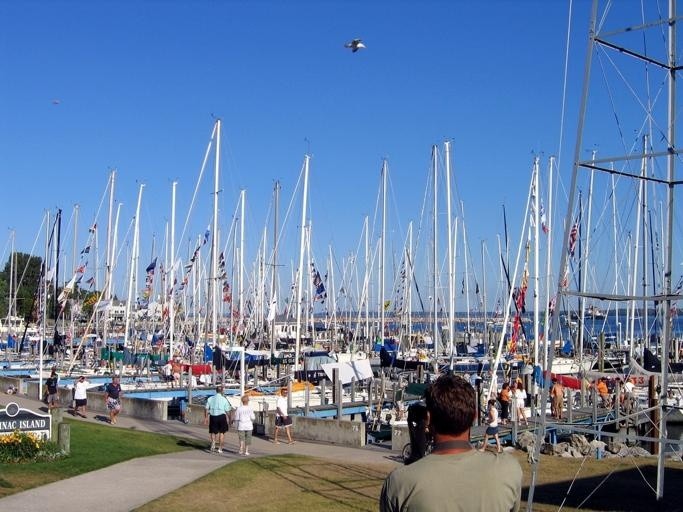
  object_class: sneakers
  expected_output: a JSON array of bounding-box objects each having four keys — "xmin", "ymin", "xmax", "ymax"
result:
[{"xmin": 239, "ymin": 450, "xmax": 250, "ymax": 456}]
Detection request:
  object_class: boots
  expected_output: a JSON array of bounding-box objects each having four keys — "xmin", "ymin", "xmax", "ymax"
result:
[{"xmin": 211, "ymin": 443, "xmax": 225, "ymax": 454}]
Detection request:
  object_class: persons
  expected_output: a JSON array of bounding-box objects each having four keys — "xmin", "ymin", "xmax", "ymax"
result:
[
  {"xmin": 500, "ymin": 381, "xmax": 529, "ymax": 426},
  {"xmin": 73, "ymin": 376, "xmax": 89, "ymax": 418},
  {"xmin": 105, "ymin": 377, "xmax": 121, "ymax": 425},
  {"xmin": 272, "ymin": 389, "xmax": 296, "ymax": 445},
  {"xmin": 550, "ymin": 378, "xmax": 564, "ymax": 420},
  {"xmin": 162, "ymin": 359, "xmax": 181, "ymax": 389},
  {"xmin": 597, "ymin": 374, "xmax": 636, "ymax": 408},
  {"xmin": 478, "ymin": 400, "xmax": 502, "ymax": 453},
  {"xmin": 379, "ymin": 375, "xmax": 522, "ymax": 512},
  {"xmin": 45, "ymin": 367, "xmax": 60, "ymax": 414},
  {"xmin": 667, "ymin": 391, "xmax": 677, "ymax": 406},
  {"xmin": 204, "ymin": 386, "xmax": 256, "ymax": 456}
]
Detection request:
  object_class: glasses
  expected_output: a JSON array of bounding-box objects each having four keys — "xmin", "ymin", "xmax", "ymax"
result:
[{"xmin": 284, "ymin": 392, "xmax": 289, "ymax": 393}]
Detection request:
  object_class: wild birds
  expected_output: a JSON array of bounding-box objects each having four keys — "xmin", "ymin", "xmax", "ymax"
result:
[{"xmin": 344, "ymin": 39, "xmax": 367, "ymax": 53}]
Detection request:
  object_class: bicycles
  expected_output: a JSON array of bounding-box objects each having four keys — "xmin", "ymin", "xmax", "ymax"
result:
[{"xmin": 402, "ymin": 443, "xmax": 427, "ymax": 461}]
